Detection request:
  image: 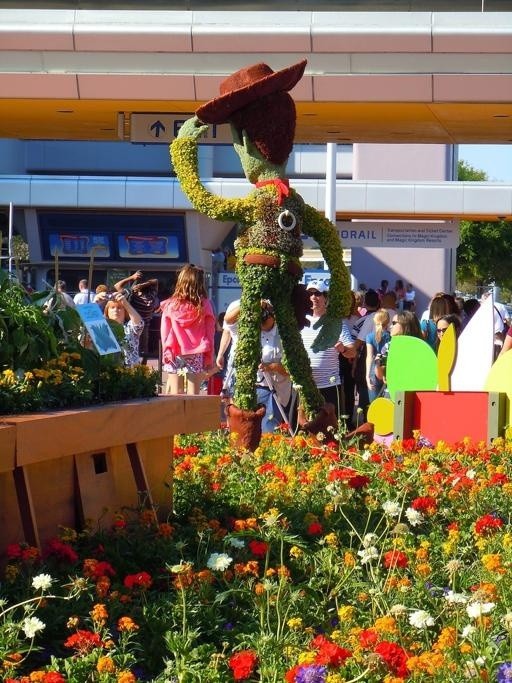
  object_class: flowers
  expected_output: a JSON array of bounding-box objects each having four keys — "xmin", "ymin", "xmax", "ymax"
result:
[{"xmin": 0, "ymin": 268, "xmax": 163, "ymax": 415}]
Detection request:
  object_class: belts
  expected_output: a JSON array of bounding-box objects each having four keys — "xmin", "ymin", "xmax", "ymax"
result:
[{"xmin": 256, "ymin": 384, "xmax": 274, "ymax": 390}]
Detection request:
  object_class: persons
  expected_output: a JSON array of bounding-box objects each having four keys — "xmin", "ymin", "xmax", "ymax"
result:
[
  {"xmin": 159, "ymin": 262, "xmax": 216, "ymax": 394},
  {"xmin": 170, "ymin": 59, "xmax": 353, "ymax": 448},
  {"xmin": 215, "ymin": 279, "xmax": 512, "ymax": 434},
  {"xmin": 40, "ymin": 271, "xmax": 158, "ymax": 369}
]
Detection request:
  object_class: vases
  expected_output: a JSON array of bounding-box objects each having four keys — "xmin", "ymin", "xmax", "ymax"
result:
[{"xmin": 1, "ymin": 394, "xmax": 221, "ymax": 567}]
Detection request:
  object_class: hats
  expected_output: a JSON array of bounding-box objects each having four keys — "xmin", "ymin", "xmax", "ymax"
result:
[{"xmin": 305, "ymin": 279, "xmax": 328, "ymax": 293}]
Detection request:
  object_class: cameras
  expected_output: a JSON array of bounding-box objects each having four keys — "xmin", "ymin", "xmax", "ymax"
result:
[
  {"xmin": 105, "ymin": 293, "xmax": 116, "ymax": 301},
  {"xmin": 261, "ymin": 308, "xmax": 270, "ymax": 321}
]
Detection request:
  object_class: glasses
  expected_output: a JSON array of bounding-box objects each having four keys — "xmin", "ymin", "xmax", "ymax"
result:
[
  {"xmin": 436, "ymin": 326, "xmax": 447, "ymax": 333},
  {"xmin": 308, "ymin": 291, "xmax": 324, "ymax": 296},
  {"xmin": 391, "ymin": 320, "xmax": 399, "ymax": 326}
]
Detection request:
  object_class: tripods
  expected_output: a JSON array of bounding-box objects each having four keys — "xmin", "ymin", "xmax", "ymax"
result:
[{"xmin": 219, "ymin": 359, "xmax": 294, "ymax": 437}]
